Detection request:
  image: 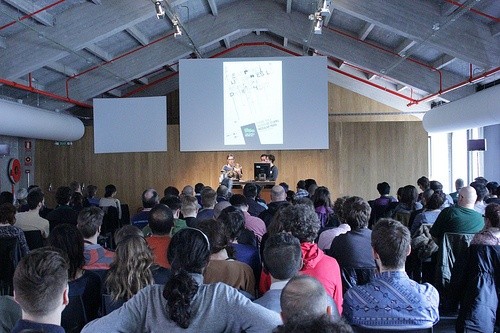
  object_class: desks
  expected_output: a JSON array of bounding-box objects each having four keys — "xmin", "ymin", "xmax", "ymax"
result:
[{"xmin": 228, "ymin": 180, "xmax": 276, "ymax": 204}]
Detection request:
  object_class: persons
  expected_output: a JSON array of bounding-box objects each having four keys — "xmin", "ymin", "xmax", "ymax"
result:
[
  {"xmin": 214, "ymin": 206, "xmax": 261, "ymax": 283},
  {"xmin": 270, "ymin": 275, "xmax": 354, "ymax": 332},
  {"xmin": 8, "ymin": 250, "xmax": 70, "ymax": 333},
  {"xmin": 219, "ymin": 154, "xmax": 242, "ymax": 182},
  {"xmin": 259, "ymin": 203, "xmax": 345, "ymax": 317},
  {"xmin": 194, "ymin": 218, "xmax": 257, "ymax": 297},
  {"xmin": 114, "ymin": 225, "xmax": 145, "ymax": 246},
  {"xmin": 101, "ymin": 233, "xmax": 169, "ymax": 316},
  {"xmin": 43, "ymin": 223, "xmax": 104, "ymax": 333},
  {"xmin": 80, "ymin": 228, "xmax": 285, "ymax": 332},
  {"xmin": 330, "ymin": 195, "xmax": 379, "ymax": 269},
  {"xmin": 265, "ymin": 154, "xmax": 278, "ymax": 180},
  {"xmin": 1, "ymin": 203, "xmax": 31, "ymax": 263},
  {"xmin": 1, "ymin": 175, "xmax": 500, "ymax": 220},
  {"xmin": 249, "ymin": 232, "xmax": 304, "ymax": 315},
  {"xmin": 318, "ymin": 196, "xmax": 354, "ymax": 250},
  {"xmin": 75, "ymin": 205, "xmax": 117, "ymax": 270},
  {"xmin": 471, "ymin": 203, "xmax": 500, "ymax": 246},
  {"xmin": 344, "ymin": 218, "xmax": 440, "ymax": 333},
  {"xmin": 430, "ymin": 185, "xmax": 485, "ymax": 238},
  {"xmin": 261, "ymin": 154, "xmax": 268, "ymax": 162},
  {"xmin": 142, "ymin": 204, "xmax": 174, "ymax": 271}
]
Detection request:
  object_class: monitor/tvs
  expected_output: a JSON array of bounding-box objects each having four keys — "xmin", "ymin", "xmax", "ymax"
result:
[{"xmin": 253, "ymin": 163, "xmax": 270, "ymax": 181}]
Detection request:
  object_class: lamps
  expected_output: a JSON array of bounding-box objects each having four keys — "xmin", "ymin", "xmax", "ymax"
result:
[
  {"xmin": 309, "ymin": 0, "xmax": 334, "ymax": 35},
  {"xmin": 153, "ymin": 0, "xmax": 185, "ymax": 38}
]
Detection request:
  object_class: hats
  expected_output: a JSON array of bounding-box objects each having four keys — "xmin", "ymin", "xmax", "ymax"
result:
[{"xmin": 16, "ymin": 188, "xmax": 28, "ymax": 199}]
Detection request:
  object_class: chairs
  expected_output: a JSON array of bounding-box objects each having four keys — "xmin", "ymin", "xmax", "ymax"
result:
[{"xmin": 0, "ymin": 202, "xmax": 500, "ymax": 333}]
